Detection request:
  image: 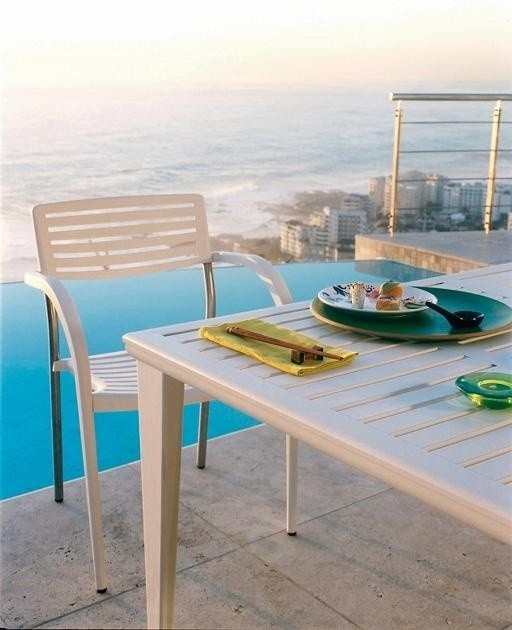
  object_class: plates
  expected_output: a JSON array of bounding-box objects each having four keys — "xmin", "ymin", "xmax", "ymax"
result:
[
  {"xmin": 318, "ymin": 282, "xmax": 438, "ymax": 315},
  {"xmin": 309, "ymin": 284, "xmax": 512, "ymax": 340}
]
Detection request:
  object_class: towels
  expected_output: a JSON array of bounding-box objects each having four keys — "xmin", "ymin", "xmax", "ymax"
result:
[{"xmin": 196, "ymin": 317, "xmax": 360, "ymax": 376}]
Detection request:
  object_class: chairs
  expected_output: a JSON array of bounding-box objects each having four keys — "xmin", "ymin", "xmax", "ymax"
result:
[{"xmin": 23, "ymin": 192, "xmax": 299, "ymax": 595}]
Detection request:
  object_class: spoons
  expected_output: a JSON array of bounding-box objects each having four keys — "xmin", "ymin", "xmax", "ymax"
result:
[{"xmin": 425, "ymin": 300, "xmax": 484, "ymax": 327}]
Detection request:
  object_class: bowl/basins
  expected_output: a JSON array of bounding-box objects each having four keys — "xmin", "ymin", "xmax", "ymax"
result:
[{"xmin": 456, "ymin": 371, "xmax": 512, "ymax": 410}]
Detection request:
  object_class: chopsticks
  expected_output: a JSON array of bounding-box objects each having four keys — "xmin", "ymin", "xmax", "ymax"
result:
[{"xmin": 226, "ymin": 325, "xmax": 343, "ymax": 362}]
